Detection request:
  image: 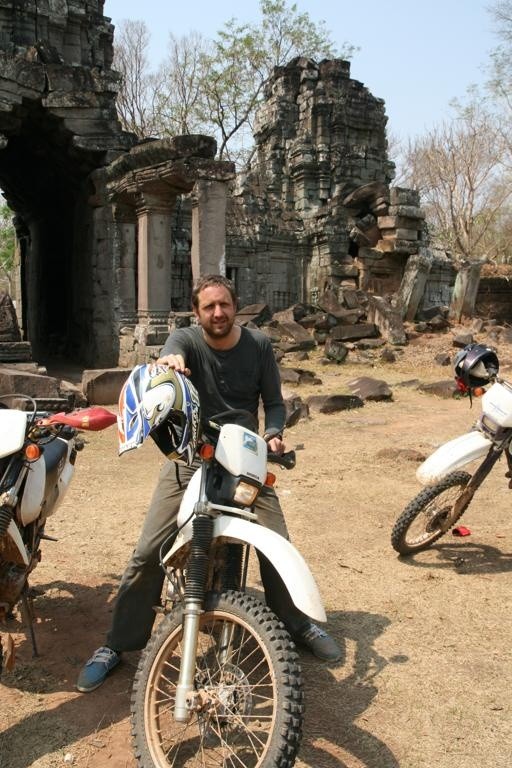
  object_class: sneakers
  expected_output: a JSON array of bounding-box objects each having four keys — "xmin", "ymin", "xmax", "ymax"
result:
[
  {"xmin": 298, "ymin": 623, "xmax": 341, "ymax": 662},
  {"xmin": 77, "ymin": 646, "xmax": 121, "ymax": 692}
]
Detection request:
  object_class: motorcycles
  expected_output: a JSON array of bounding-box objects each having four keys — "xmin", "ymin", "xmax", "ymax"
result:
[
  {"xmin": 390, "ymin": 360, "xmax": 512, "ymax": 556},
  {"xmin": 130, "ymin": 408, "xmax": 328, "ymax": 768},
  {"xmin": 0, "ymin": 394, "xmax": 117, "ymax": 684}
]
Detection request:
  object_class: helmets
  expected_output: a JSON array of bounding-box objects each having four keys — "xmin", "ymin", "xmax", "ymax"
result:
[
  {"xmin": 117, "ymin": 364, "xmax": 202, "ymax": 467},
  {"xmin": 453, "ymin": 343, "xmax": 499, "ymax": 388}
]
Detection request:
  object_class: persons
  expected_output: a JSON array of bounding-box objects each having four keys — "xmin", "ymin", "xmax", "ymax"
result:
[{"xmin": 76, "ymin": 274, "xmax": 343, "ymax": 693}]
